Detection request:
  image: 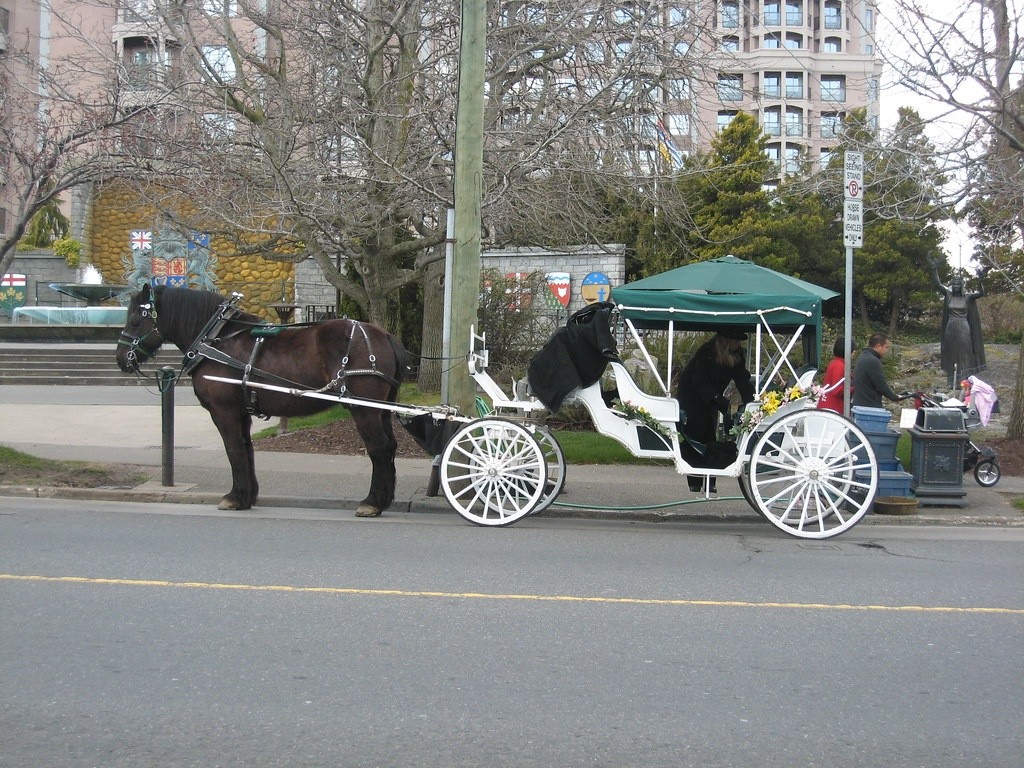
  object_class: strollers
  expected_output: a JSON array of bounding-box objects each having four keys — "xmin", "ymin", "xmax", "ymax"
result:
[{"xmin": 899, "ymin": 391, "xmax": 1002, "ymax": 487}]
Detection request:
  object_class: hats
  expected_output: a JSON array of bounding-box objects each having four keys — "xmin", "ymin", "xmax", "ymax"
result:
[{"xmin": 717, "ymin": 325, "xmax": 748, "ymax": 341}]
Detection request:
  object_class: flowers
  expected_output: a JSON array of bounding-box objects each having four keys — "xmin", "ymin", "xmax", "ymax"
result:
[
  {"xmin": 729, "ymin": 383, "xmax": 827, "ymax": 437},
  {"xmin": 610, "ymin": 397, "xmax": 684, "ymax": 442}
]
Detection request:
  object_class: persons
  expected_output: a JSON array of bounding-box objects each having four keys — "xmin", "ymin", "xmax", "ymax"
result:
[
  {"xmin": 926, "ymin": 257, "xmax": 987, "ymax": 390},
  {"xmin": 853, "ymin": 333, "xmax": 899, "ymax": 408},
  {"xmin": 817, "ymin": 337, "xmax": 858, "ymax": 414},
  {"xmin": 677, "ymin": 325, "xmax": 756, "ymax": 493}
]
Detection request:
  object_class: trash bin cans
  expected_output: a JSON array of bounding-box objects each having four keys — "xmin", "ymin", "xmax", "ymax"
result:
[{"xmin": 907, "ymin": 408, "xmax": 970, "ymax": 508}]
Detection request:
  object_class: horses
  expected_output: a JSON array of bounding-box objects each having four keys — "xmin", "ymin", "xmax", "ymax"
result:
[{"xmin": 115, "ymin": 283, "xmax": 409, "ymax": 517}]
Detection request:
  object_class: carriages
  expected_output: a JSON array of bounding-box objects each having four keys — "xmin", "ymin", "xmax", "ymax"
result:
[{"xmin": 114, "ymin": 253, "xmax": 879, "ymax": 540}]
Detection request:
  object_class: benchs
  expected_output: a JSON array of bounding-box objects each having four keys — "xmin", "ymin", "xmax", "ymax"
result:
[
  {"xmin": 744, "ymin": 363, "xmax": 818, "ymax": 433},
  {"xmin": 610, "ymin": 361, "xmax": 680, "ymax": 422}
]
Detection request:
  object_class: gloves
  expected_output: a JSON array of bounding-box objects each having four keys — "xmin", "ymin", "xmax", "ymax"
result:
[{"xmin": 714, "ymin": 394, "xmax": 729, "ymax": 414}]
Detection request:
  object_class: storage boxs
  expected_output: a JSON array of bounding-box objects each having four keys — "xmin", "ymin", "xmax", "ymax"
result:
[
  {"xmin": 855, "ymin": 470, "xmax": 914, "ymax": 497},
  {"xmin": 851, "ymin": 406, "xmax": 892, "ymax": 432},
  {"xmin": 848, "ymin": 428, "xmax": 901, "ymax": 460},
  {"xmin": 853, "ymin": 457, "xmax": 901, "ymax": 471}
]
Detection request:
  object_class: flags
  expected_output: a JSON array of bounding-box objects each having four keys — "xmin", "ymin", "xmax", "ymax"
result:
[{"xmin": 656, "ymin": 114, "xmax": 685, "ymax": 176}]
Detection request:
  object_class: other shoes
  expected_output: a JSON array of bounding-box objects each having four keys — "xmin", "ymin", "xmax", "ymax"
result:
[
  {"xmin": 710, "ymin": 487, "xmax": 718, "ymax": 492},
  {"xmin": 690, "ymin": 485, "xmax": 701, "ymax": 492}
]
